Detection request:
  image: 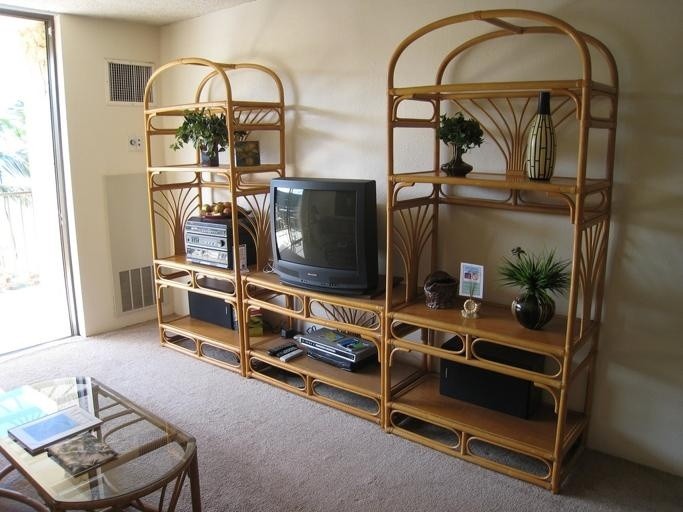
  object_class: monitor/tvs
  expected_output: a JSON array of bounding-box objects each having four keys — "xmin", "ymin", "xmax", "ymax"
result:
[{"xmin": 271, "ymin": 176, "xmax": 377, "ymax": 297}]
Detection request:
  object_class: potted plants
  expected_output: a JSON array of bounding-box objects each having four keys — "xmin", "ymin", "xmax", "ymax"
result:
[
  {"xmin": 490, "ymin": 244, "xmax": 581, "ymax": 331},
  {"xmin": 437, "ymin": 110, "xmax": 484, "ymax": 178},
  {"xmin": 168, "ymin": 107, "xmax": 252, "ymax": 168}
]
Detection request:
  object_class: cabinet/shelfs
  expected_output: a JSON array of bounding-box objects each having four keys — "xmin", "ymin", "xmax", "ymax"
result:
[
  {"xmin": 383, "ymin": 10, "xmax": 617, "ymax": 496},
  {"xmin": 143, "ymin": 57, "xmax": 286, "ymax": 377},
  {"xmin": 240, "ymin": 269, "xmax": 427, "ymax": 426}
]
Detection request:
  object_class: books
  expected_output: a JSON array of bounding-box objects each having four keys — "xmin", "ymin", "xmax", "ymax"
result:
[
  {"xmin": 46, "ymin": 430, "xmax": 119, "ymax": 478},
  {"xmin": 318, "ymin": 330, "xmax": 345, "ymax": 342},
  {"xmin": 6, "ymin": 403, "xmax": 104, "ymax": 452},
  {"xmin": 337, "ymin": 336, "xmax": 370, "ymax": 352}
]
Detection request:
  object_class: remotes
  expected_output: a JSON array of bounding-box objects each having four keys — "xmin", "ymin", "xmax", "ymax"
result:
[
  {"xmin": 280, "ymin": 349, "xmax": 305, "ymax": 360},
  {"xmin": 266, "ymin": 341, "xmax": 297, "ymax": 354},
  {"xmin": 276, "ymin": 347, "xmax": 297, "ymax": 355}
]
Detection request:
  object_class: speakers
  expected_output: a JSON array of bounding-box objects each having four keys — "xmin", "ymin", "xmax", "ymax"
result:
[
  {"xmin": 441, "ymin": 335, "xmax": 544, "ymax": 419},
  {"xmin": 187, "ymin": 277, "xmax": 233, "ymax": 329}
]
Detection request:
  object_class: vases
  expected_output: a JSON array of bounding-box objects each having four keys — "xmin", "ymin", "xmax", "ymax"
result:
[{"xmin": 526, "ymin": 90, "xmax": 558, "ymax": 183}]
